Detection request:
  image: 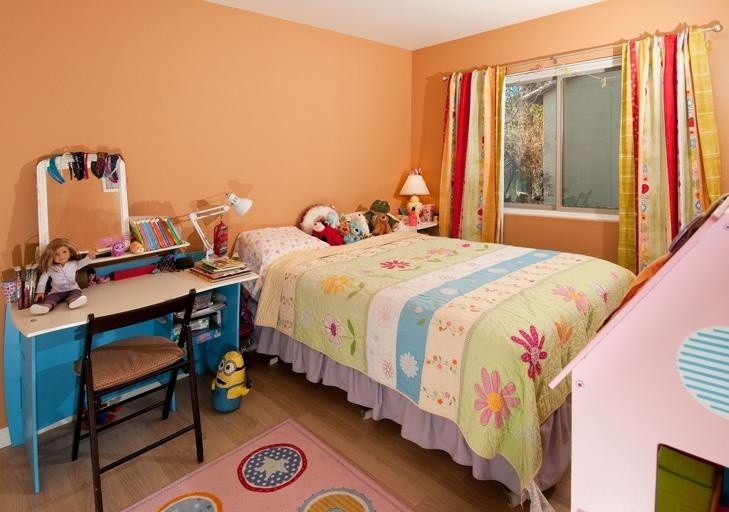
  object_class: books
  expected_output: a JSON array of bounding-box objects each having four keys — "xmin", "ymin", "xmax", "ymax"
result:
[
  {"xmin": 128, "ymin": 216, "xmax": 182, "ymax": 254},
  {"xmin": 189, "ymin": 256, "xmax": 252, "ymax": 283}
]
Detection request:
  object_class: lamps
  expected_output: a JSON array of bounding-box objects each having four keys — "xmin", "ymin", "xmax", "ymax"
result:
[
  {"xmin": 397, "ymin": 169, "xmax": 430, "ymax": 214},
  {"xmin": 185, "ymin": 192, "xmax": 253, "ymax": 258}
]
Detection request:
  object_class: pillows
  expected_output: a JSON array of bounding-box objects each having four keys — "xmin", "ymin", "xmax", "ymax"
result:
[{"xmin": 231, "ymin": 224, "xmax": 330, "ymax": 275}]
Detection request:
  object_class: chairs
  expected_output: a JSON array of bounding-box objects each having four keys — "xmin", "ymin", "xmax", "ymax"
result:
[{"xmin": 64, "ymin": 286, "xmax": 206, "ymax": 512}]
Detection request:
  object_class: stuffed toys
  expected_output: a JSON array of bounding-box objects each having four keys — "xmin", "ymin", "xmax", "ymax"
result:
[
  {"xmin": 210, "ymin": 350, "xmax": 253, "ymax": 415},
  {"xmin": 300, "ymin": 196, "xmax": 400, "ymax": 247},
  {"xmin": 98, "ymin": 232, "xmax": 133, "ymax": 258}
]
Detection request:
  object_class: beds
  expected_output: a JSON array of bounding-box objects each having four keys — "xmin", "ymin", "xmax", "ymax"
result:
[{"xmin": 243, "ymin": 228, "xmax": 638, "ymax": 505}]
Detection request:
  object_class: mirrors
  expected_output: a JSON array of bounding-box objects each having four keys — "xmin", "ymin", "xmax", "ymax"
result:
[{"xmin": 35, "ymin": 153, "xmax": 130, "ymax": 260}]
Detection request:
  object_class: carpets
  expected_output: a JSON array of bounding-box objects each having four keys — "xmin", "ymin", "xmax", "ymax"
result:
[{"xmin": 117, "ymin": 418, "xmax": 416, "ymax": 512}]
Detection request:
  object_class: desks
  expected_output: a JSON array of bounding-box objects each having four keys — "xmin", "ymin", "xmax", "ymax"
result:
[
  {"xmin": 1, "ymin": 260, "xmax": 261, "ymax": 494},
  {"xmin": 405, "ymin": 220, "xmax": 437, "ymax": 234}
]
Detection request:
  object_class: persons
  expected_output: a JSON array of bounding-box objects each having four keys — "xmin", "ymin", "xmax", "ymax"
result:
[{"xmin": 30, "ymin": 237, "xmax": 98, "ymax": 315}]
